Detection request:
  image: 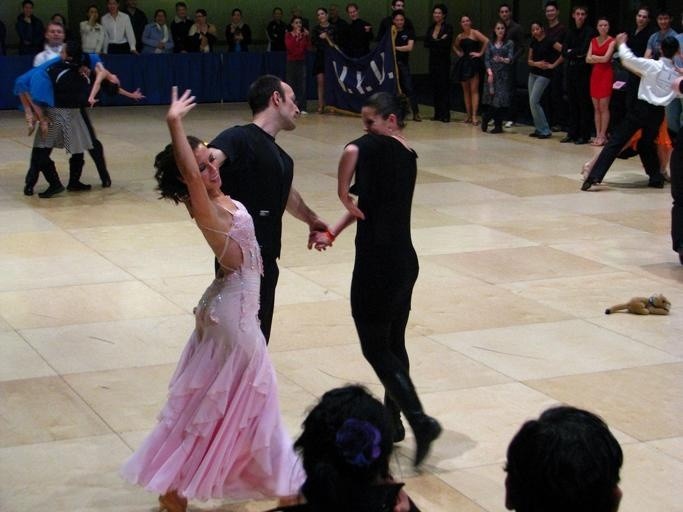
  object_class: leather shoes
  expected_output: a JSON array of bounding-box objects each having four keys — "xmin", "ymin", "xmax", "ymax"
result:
[
  {"xmin": 414, "ymin": 419, "xmax": 442, "ymax": 467},
  {"xmin": 393, "ymin": 424, "xmax": 407, "ymax": 444}
]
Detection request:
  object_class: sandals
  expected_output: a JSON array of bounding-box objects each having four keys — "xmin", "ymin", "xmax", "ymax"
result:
[
  {"xmin": 590, "ymin": 136, "xmax": 608, "ymax": 146},
  {"xmin": 26, "ymin": 113, "xmax": 38, "ymax": 137}
]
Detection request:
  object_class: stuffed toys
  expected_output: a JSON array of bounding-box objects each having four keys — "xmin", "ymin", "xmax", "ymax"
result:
[{"xmin": 606, "ymin": 294, "xmax": 671, "ymax": 315}]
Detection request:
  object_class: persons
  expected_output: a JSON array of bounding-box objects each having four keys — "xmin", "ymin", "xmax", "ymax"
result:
[
  {"xmin": 266, "ymin": 385, "xmax": 421, "ymax": 512},
  {"xmin": 205, "ymin": 73, "xmax": 327, "ymax": 345},
  {"xmin": 309, "ymin": 90, "xmax": 441, "ymax": 466},
  {"xmin": 119, "ymin": 84, "xmax": 307, "ymax": 512},
  {"xmin": 503, "ymin": 407, "xmax": 623, "ymax": 512}
]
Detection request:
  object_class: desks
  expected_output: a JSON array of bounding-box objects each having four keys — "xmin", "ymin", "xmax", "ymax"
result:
[
  {"xmin": 2, "ymin": 52, "xmax": 36, "ymax": 110},
  {"xmin": 97, "ymin": 52, "xmax": 319, "ymax": 107}
]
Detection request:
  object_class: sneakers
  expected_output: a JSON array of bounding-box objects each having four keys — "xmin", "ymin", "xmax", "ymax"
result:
[
  {"xmin": 300, "ymin": 105, "xmax": 336, "ymax": 116},
  {"xmin": 66, "ymin": 182, "xmax": 92, "ymax": 191},
  {"xmin": 412, "ymin": 113, "xmax": 421, "ymax": 122},
  {"xmin": 24, "ymin": 183, "xmax": 33, "ymax": 196},
  {"xmin": 647, "ymin": 172, "xmax": 671, "ymax": 187},
  {"xmin": 38, "ymin": 184, "xmax": 65, "ymax": 198},
  {"xmin": 559, "ymin": 134, "xmax": 575, "ymax": 142},
  {"xmin": 575, "ymin": 136, "xmax": 590, "ymax": 145},
  {"xmin": 430, "ymin": 114, "xmax": 514, "ymax": 132},
  {"xmin": 529, "ymin": 129, "xmax": 552, "ymax": 139},
  {"xmin": 581, "ymin": 163, "xmax": 596, "ymax": 190},
  {"xmin": 101, "ymin": 175, "xmax": 112, "ymax": 188}
]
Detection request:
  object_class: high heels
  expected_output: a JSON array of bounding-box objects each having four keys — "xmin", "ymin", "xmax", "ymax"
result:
[
  {"xmin": 157, "ymin": 491, "xmax": 188, "ymax": 512},
  {"xmin": 40, "ymin": 116, "xmax": 53, "ymax": 141}
]
